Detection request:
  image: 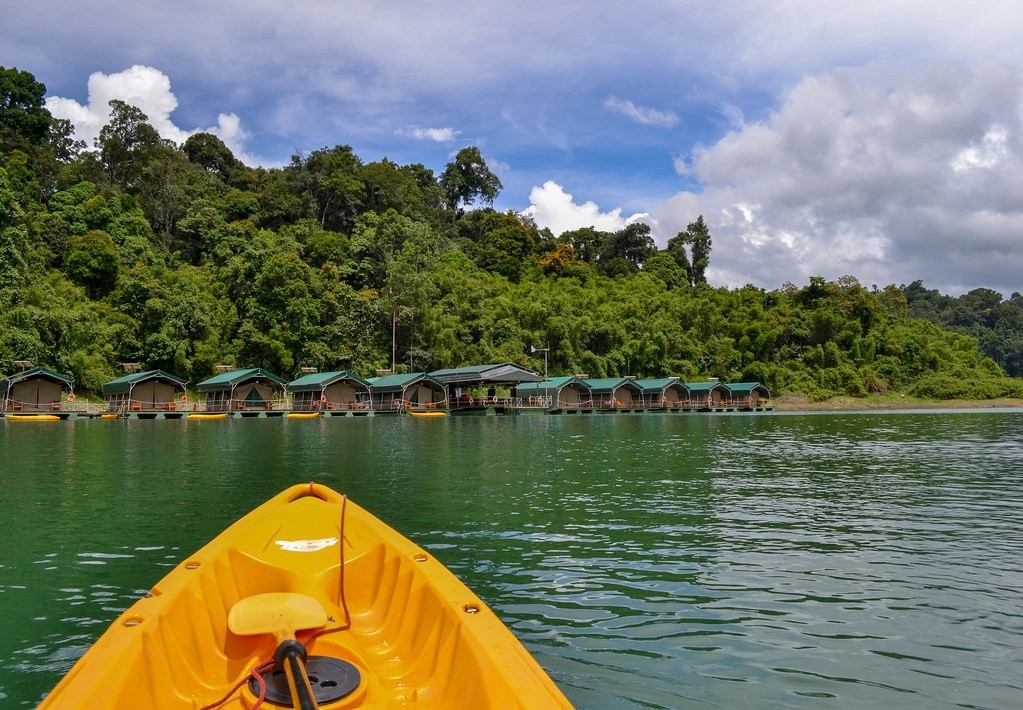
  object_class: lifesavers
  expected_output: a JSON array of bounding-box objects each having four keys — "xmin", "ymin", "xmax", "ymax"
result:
[
  {"xmin": 67, "ymin": 394, "xmax": 75, "ymax": 402},
  {"xmin": 181, "ymin": 396, "xmax": 188, "ymax": 403},
  {"xmin": 321, "ymin": 396, "xmax": 326, "ymax": 403},
  {"xmin": 492, "ymin": 396, "xmax": 498, "ymax": 403},
  {"xmin": 468, "ymin": 398, "xmax": 473, "ymax": 403}
]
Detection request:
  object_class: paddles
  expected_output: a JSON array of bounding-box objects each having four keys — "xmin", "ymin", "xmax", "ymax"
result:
[{"xmin": 228, "ymin": 592, "xmax": 329, "ymax": 710}]
